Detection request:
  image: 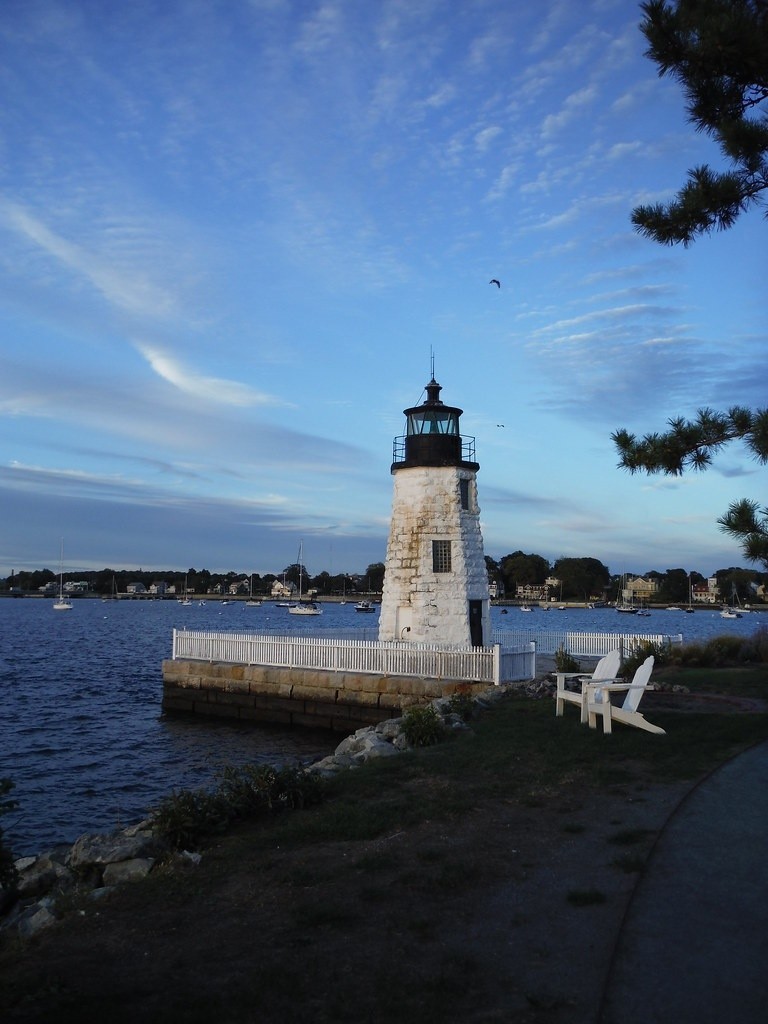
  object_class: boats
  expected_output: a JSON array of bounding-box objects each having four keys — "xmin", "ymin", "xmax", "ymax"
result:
[
  {"xmin": 177, "ymin": 574, "xmax": 262, "ymax": 607},
  {"xmin": 354, "ymin": 599, "xmax": 376, "ymax": 613},
  {"xmin": 501, "ymin": 600, "xmax": 763, "ymax": 621},
  {"xmin": 340, "ymin": 600, "xmax": 347, "ymax": 604},
  {"xmin": 275, "ymin": 599, "xmax": 298, "ymax": 608}
]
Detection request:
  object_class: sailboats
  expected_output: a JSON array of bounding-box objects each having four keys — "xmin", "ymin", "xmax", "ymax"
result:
[
  {"xmin": 102, "ymin": 575, "xmax": 119, "ymax": 603},
  {"xmin": 50, "ymin": 537, "xmax": 74, "ymax": 610},
  {"xmin": 289, "ymin": 539, "xmax": 324, "ymax": 615}
]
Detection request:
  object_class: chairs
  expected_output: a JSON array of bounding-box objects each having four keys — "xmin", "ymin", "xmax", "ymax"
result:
[
  {"xmin": 586, "ymin": 655, "xmax": 667, "ymax": 734},
  {"xmin": 550, "ymin": 649, "xmax": 624, "ymax": 723}
]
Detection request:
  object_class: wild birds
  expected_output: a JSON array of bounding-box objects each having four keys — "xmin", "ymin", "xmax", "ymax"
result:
[{"xmin": 488, "ymin": 279, "xmax": 500, "ymax": 288}]
